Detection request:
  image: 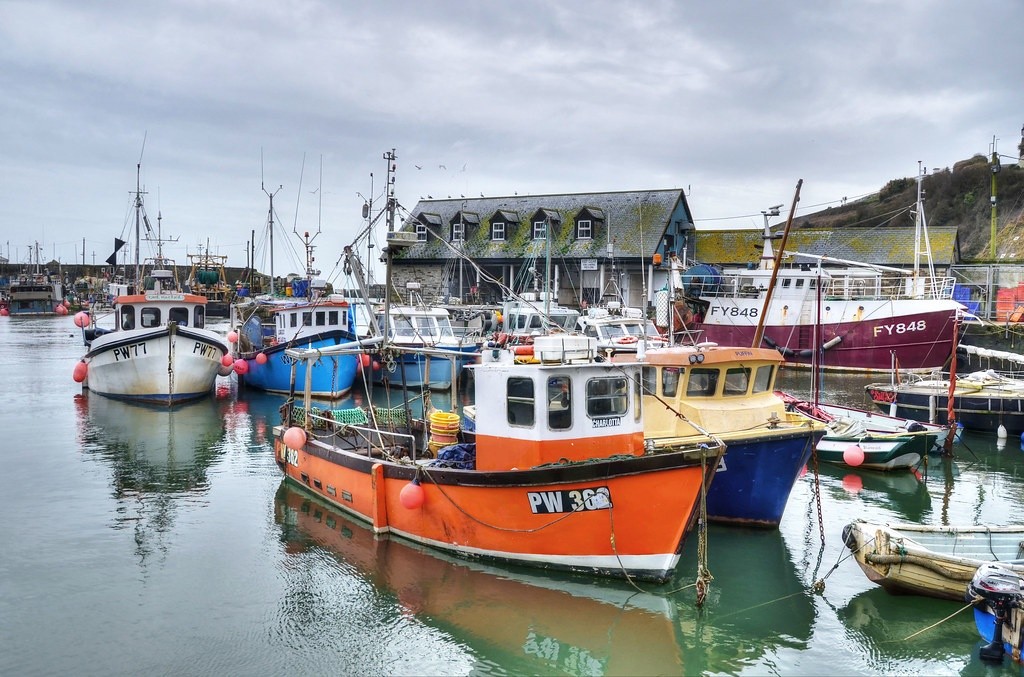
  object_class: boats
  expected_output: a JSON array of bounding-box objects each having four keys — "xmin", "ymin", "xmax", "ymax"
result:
[
  {"xmin": 864, "ymin": 368, "xmax": 1024, "ymax": 438},
  {"xmin": 964, "ymin": 558, "xmax": 1024, "ymax": 668},
  {"xmin": 840, "ymin": 516, "xmax": 1024, "ymax": 603},
  {"xmin": 7, "ymin": 125, "xmax": 969, "ymax": 587}
]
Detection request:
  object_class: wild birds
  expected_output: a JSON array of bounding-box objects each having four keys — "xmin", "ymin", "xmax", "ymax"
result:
[{"xmin": 415, "ymin": 162, "xmax": 469, "ymax": 173}]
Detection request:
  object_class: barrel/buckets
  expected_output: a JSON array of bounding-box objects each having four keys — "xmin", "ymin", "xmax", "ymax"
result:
[
  {"xmin": 428, "ymin": 410, "xmax": 460, "ymax": 459},
  {"xmin": 684, "ymin": 265, "xmax": 719, "ymax": 295}
]
